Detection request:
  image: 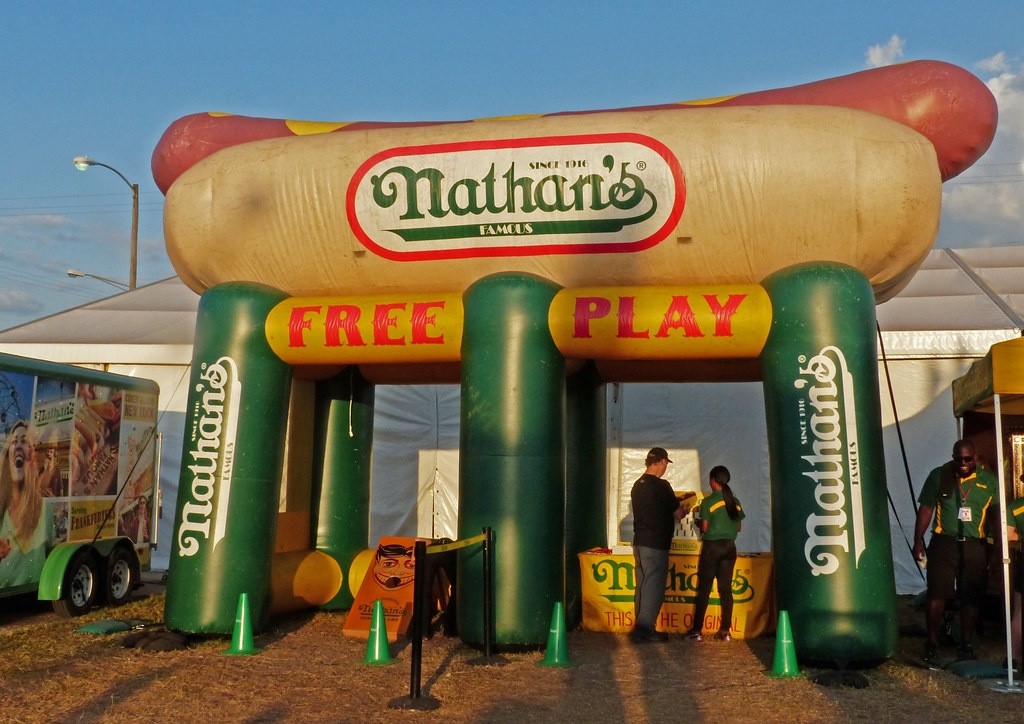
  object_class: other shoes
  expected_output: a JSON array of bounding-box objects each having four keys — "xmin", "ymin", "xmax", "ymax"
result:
[
  {"xmin": 1003, "ymin": 657, "xmax": 1018, "ymax": 673},
  {"xmin": 924, "ymin": 641, "xmax": 938, "ymax": 662},
  {"xmin": 632, "ymin": 628, "xmax": 669, "ymax": 643},
  {"xmin": 956, "ymin": 641, "xmax": 975, "ymax": 660},
  {"xmin": 680, "ymin": 630, "xmax": 704, "ymax": 642},
  {"xmin": 713, "ymin": 630, "xmax": 732, "ymax": 642}
]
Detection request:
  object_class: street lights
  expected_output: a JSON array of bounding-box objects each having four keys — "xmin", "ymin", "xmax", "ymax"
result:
[{"xmin": 67, "ymin": 153, "xmax": 141, "ymax": 295}]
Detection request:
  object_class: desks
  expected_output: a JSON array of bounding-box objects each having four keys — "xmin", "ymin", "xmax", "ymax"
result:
[{"xmin": 578, "ymin": 547, "xmax": 777, "ymax": 640}]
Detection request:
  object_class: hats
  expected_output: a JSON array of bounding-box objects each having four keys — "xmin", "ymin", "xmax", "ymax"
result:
[{"xmin": 646, "ymin": 447, "xmax": 673, "ymax": 463}]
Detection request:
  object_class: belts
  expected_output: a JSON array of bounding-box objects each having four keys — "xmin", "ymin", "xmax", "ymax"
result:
[{"xmin": 932, "ymin": 532, "xmax": 980, "ymax": 541}]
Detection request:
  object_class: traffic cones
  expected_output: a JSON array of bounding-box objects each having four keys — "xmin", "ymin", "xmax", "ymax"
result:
[
  {"xmin": 534, "ymin": 602, "xmax": 584, "ymax": 670},
  {"xmin": 353, "ymin": 600, "xmax": 402, "ymax": 667},
  {"xmin": 761, "ymin": 609, "xmax": 806, "ymax": 680},
  {"xmin": 217, "ymin": 592, "xmax": 264, "ymax": 656}
]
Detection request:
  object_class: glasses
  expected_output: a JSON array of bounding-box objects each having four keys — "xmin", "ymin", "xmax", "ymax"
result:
[
  {"xmin": 952, "ymin": 453, "xmax": 975, "ymax": 462},
  {"xmin": 138, "ymin": 501, "xmax": 146, "ymax": 505}
]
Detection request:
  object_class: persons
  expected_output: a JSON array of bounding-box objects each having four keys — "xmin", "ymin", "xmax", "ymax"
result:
[
  {"xmin": 631, "ymin": 448, "xmax": 691, "ymax": 642},
  {"xmin": 681, "ymin": 465, "xmax": 745, "ymax": 642},
  {"xmin": 912, "ymin": 440, "xmax": 1003, "ymax": 664},
  {"xmin": 1004, "ymin": 470, "xmax": 1024, "ymax": 674},
  {"xmin": 1, "ymin": 418, "xmax": 150, "ymax": 591}
]
